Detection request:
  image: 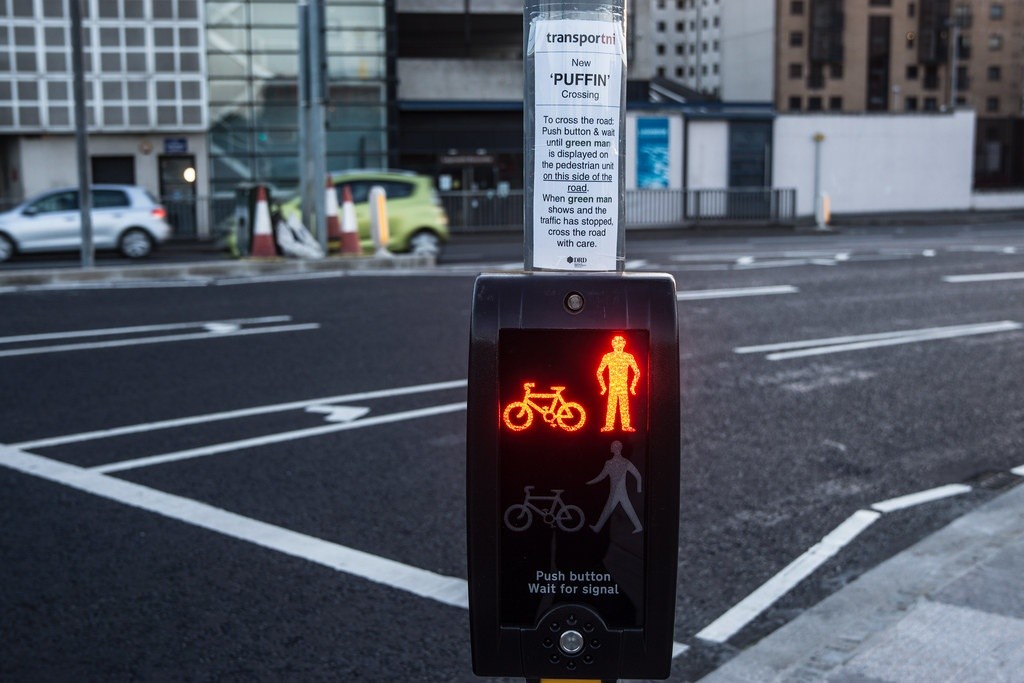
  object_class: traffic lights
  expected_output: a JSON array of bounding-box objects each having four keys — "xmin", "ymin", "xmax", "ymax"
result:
[{"xmin": 468, "ymin": 272, "xmax": 681, "ymax": 676}]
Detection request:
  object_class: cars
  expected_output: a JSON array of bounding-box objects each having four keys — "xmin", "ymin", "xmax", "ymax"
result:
[
  {"xmin": 1, "ymin": 184, "xmax": 172, "ymax": 263},
  {"xmin": 229, "ymin": 166, "xmax": 451, "ymax": 269}
]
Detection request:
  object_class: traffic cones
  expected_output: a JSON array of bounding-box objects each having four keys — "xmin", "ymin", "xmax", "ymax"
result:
[
  {"xmin": 249, "ymin": 186, "xmax": 283, "ymax": 265},
  {"xmin": 324, "ymin": 176, "xmax": 345, "ymax": 245},
  {"xmin": 335, "ymin": 186, "xmax": 366, "ymax": 260}
]
